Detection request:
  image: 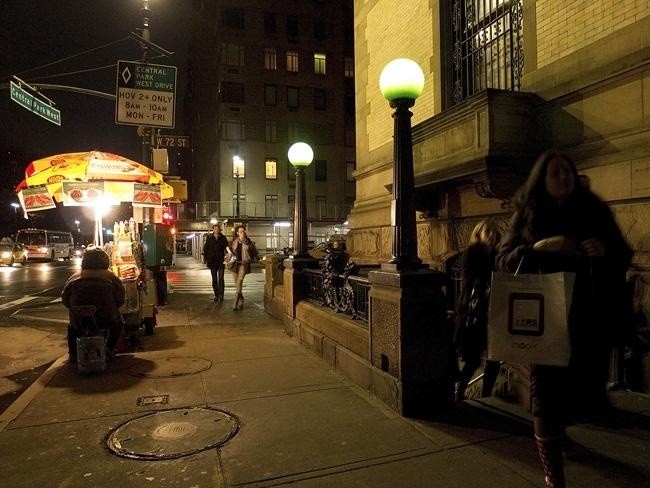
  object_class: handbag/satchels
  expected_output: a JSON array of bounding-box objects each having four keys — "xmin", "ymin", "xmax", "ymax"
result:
[{"xmin": 487, "ymin": 269, "xmax": 574, "ymax": 367}]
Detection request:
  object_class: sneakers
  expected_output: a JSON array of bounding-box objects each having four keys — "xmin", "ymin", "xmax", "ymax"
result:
[
  {"xmin": 214, "ymin": 295, "xmax": 223, "ymax": 301},
  {"xmin": 233, "ymin": 296, "xmax": 244, "ymax": 310}
]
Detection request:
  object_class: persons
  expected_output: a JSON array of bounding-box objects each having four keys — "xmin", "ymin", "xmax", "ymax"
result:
[
  {"xmin": 232, "ymin": 226, "xmax": 260, "ymax": 310},
  {"xmin": 495, "ymin": 149, "xmax": 635, "ymax": 488},
  {"xmin": 176, "ymin": 243, "xmax": 192, "ymax": 255},
  {"xmin": 62, "ymin": 247, "xmax": 127, "ymax": 362},
  {"xmin": 202, "ymin": 224, "xmax": 234, "ymax": 302},
  {"xmin": 453, "ymin": 220, "xmax": 501, "ymax": 403}
]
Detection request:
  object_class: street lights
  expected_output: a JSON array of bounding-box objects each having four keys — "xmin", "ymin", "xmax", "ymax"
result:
[
  {"xmin": 233, "ymin": 154, "xmax": 245, "ymax": 218},
  {"xmin": 11, "ymin": 203, "xmax": 20, "ymax": 213},
  {"xmin": 378, "ymin": 56, "xmax": 430, "ymax": 270},
  {"xmin": 75, "ymin": 220, "xmax": 80, "ymax": 228},
  {"xmin": 288, "ymin": 140, "xmax": 317, "ymax": 267}
]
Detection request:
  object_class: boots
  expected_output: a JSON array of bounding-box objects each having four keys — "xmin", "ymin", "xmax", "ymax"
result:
[{"xmin": 535, "ymin": 435, "xmax": 565, "ymax": 488}]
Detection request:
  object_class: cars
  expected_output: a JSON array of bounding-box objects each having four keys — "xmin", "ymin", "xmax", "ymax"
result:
[
  {"xmin": 0, "ymin": 242, "xmax": 27, "ymax": 267},
  {"xmin": 258, "ymin": 254, "xmax": 290, "ymax": 270},
  {"xmin": 308, "ymin": 235, "xmax": 347, "ymax": 259}
]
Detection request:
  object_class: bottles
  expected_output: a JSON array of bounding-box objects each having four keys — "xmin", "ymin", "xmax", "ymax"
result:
[
  {"xmin": 113, "ymin": 216, "xmax": 137, "ymax": 239},
  {"xmin": 112, "ymin": 243, "xmax": 121, "ymax": 260}
]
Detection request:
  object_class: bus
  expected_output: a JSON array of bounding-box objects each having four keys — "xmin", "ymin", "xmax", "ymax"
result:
[{"xmin": 15, "ymin": 228, "xmax": 75, "ymax": 262}]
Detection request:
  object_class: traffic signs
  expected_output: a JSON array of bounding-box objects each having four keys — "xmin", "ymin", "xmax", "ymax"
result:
[
  {"xmin": 10, "ymin": 80, "xmax": 61, "ymax": 126},
  {"xmin": 155, "ymin": 134, "xmax": 190, "ymax": 148}
]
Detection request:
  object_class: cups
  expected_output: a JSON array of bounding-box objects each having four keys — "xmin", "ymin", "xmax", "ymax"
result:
[{"xmin": 111, "ymin": 266, "xmax": 120, "ymax": 277}]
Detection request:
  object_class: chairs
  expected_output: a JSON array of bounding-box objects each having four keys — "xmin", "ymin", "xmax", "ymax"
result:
[{"xmin": 69, "ymin": 305, "xmax": 115, "ymax": 359}]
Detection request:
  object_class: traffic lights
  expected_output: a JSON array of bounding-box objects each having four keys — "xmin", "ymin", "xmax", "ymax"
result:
[{"xmin": 150, "ymin": 146, "xmax": 169, "ymax": 173}]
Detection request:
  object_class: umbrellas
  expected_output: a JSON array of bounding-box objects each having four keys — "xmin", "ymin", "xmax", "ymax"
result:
[{"xmin": 16, "ymin": 148, "xmax": 175, "ymax": 251}]
